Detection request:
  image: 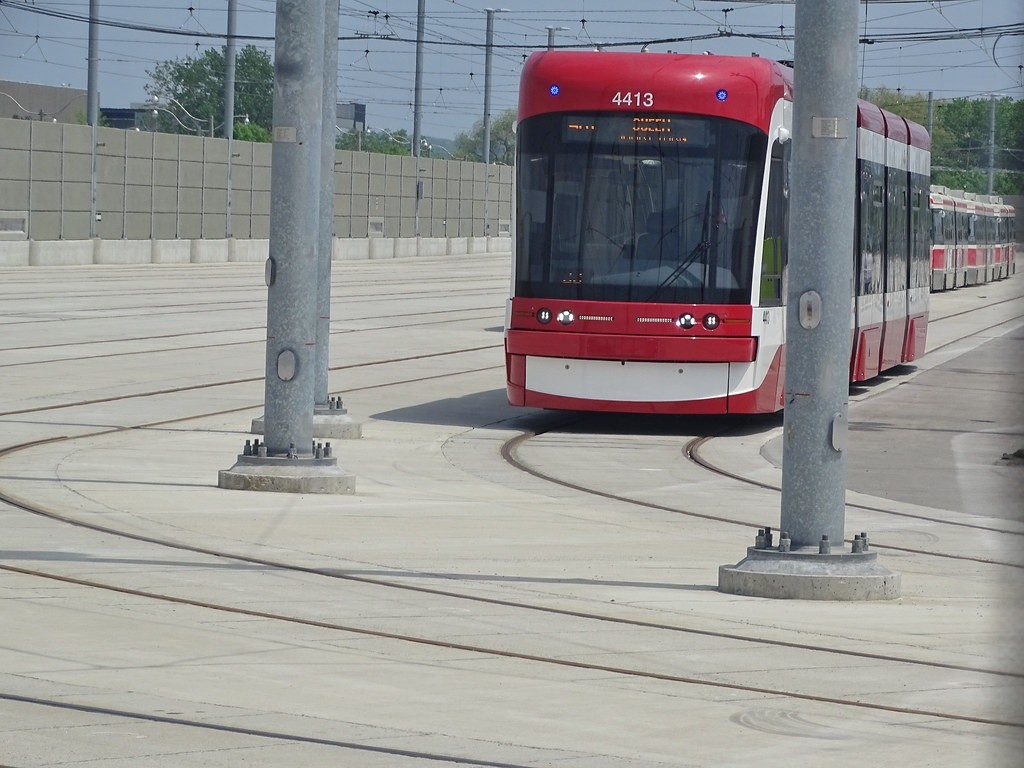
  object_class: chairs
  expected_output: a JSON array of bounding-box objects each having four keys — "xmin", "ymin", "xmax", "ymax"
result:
[{"xmin": 635, "ymin": 212, "xmax": 682, "ymax": 260}]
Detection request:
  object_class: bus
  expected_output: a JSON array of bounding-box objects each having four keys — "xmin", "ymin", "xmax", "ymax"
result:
[
  {"xmin": 501, "ymin": 48, "xmax": 935, "ymax": 416},
  {"xmin": 929, "ymin": 184, "xmax": 1015, "ymax": 293}
]
